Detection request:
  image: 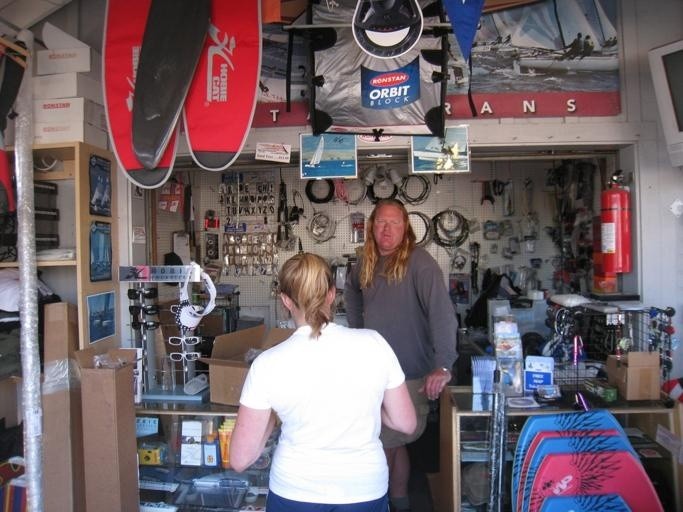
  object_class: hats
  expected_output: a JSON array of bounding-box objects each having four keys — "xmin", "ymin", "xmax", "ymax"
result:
[
  {"xmin": 176, "ymin": 261, "xmax": 216, "ymax": 330},
  {"xmin": 461, "ymin": 462, "xmax": 508, "ymax": 506}
]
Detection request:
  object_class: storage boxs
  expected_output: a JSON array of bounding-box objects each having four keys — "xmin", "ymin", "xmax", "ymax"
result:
[
  {"xmin": 40, "ymin": 300, "xmax": 138, "ymax": 512},
  {"xmin": 197, "ymin": 320, "xmax": 296, "ymax": 406},
  {"xmin": 602, "ymin": 347, "xmax": 661, "ymax": 403}
]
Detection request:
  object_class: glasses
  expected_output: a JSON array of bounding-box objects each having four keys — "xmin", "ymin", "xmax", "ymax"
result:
[
  {"xmin": 169, "ymin": 336, "xmax": 202, "ymax": 346},
  {"xmin": 170, "ymin": 305, "xmax": 201, "ymax": 313},
  {"xmin": 132, "ymin": 321, "xmax": 159, "ymax": 330},
  {"xmin": 128, "ymin": 288, "xmax": 157, "ymax": 299},
  {"xmin": 129, "ymin": 305, "xmax": 159, "ymax": 316},
  {"xmin": 555, "ymin": 307, "xmax": 574, "ymax": 335},
  {"xmin": 170, "ymin": 352, "xmax": 201, "ymax": 361}
]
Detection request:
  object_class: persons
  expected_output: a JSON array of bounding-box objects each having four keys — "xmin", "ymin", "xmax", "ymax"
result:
[
  {"xmin": 344, "ymin": 197, "xmax": 462, "ymax": 511},
  {"xmin": 229, "ymin": 253, "xmax": 416, "ymax": 510}
]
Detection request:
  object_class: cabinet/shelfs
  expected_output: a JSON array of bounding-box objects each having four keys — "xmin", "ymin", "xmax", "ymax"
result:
[
  {"xmin": 440, "ymin": 386, "xmax": 676, "ymax": 511},
  {"xmin": 2, "ymin": 141, "xmax": 118, "ymax": 388},
  {"xmin": 133, "ymin": 406, "xmax": 283, "ymax": 512}
]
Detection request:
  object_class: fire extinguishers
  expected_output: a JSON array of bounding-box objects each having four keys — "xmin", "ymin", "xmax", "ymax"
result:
[{"xmin": 600, "ymin": 169, "xmax": 634, "ymax": 273}]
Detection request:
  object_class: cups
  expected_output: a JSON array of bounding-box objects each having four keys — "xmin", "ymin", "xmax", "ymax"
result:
[{"xmin": 203, "ymin": 432, "xmax": 220, "ymax": 467}]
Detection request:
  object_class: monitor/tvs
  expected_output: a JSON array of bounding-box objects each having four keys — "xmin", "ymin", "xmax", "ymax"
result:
[{"xmin": 646, "ymin": 37, "xmax": 683, "ymax": 170}]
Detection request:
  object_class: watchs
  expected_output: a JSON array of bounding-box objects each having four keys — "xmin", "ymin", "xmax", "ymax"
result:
[{"xmin": 438, "ymin": 364, "xmax": 450, "ymax": 381}]
